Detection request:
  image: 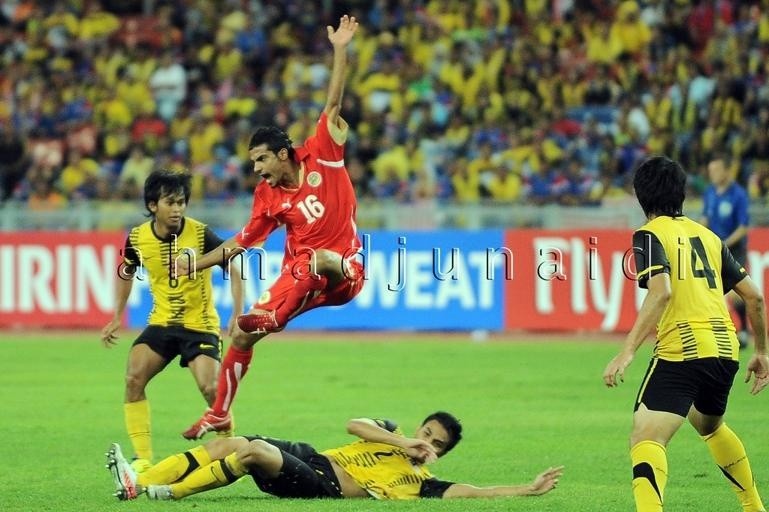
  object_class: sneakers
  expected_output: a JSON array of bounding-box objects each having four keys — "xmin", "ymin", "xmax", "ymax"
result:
[
  {"xmin": 106, "ymin": 443, "xmax": 138, "ymax": 500},
  {"xmin": 237, "ymin": 310, "xmax": 285, "ymax": 336},
  {"xmin": 183, "ymin": 410, "xmax": 233, "ymax": 438},
  {"xmin": 146, "ymin": 485, "xmax": 174, "ymax": 501}
]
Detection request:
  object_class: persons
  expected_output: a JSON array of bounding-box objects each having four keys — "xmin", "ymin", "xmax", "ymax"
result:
[
  {"xmin": 167, "ymin": 15, "xmax": 365, "ymax": 440},
  {"xmin": 603, "ymin": 157, "xmax": 769, "ymax": 512},
  {"xmin": 0, "ymin": 0, "xmax": 769, "ymax": 206},
  {"xmin": 100, "ymin": 170, "xmax": 244, "ymax": 472},
  {"xmin": 104, "ymin": 412, "xmax": 565, "ymax": 501},
  {"xmin": 700, "ymin": 152, "xmax": 750, "ymax": 349}
]
